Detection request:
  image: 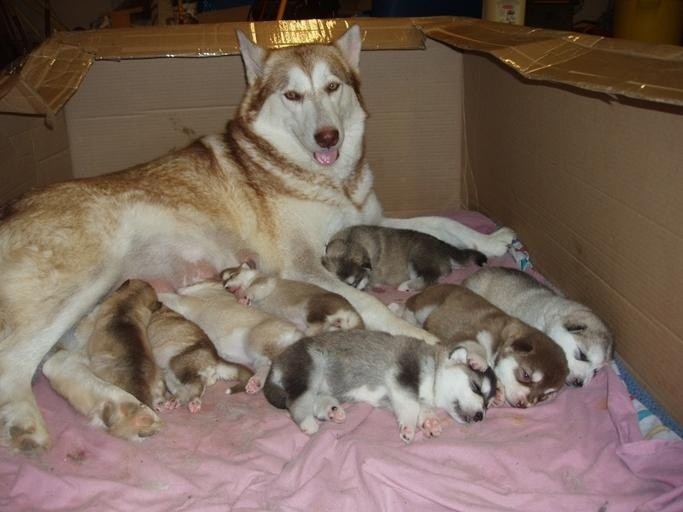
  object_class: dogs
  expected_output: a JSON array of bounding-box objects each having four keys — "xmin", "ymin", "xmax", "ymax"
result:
[
  {"xmin": 263, "ymin": 328, "xmax": 499, "ymax": 446},
  {"xmin": 155, "ymin": 278, "xmax": 306, "ymax": 395},
  {"xmin": 218, "ymin": 260, "xmax": 366, "ymax": 338},
  {"xmin": 386, "ymin": 282, "xmax": 570, "ymax": 409},
  {"xmin": 146, "ymin": 300, "xmax": 256, "ymax": 415},
  {"xmin": 321, "ymin": 225, "xmax": 488, "ymax": 294},
  {"xmin": 74, "ymin": 278, "xmax": 167, "ymax": 414},
  {"xmin": 460, "ymin": 264, "xmax": 614, "ymax": 388},
  {"xmin": 0, "ymin": 23, "xmax": 518, "ymax": 454}
]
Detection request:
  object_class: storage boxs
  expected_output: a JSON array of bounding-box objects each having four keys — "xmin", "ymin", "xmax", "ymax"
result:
[{"xmin": 0, "ymin": 15, "xmax": 683, "ymax": 512}]
